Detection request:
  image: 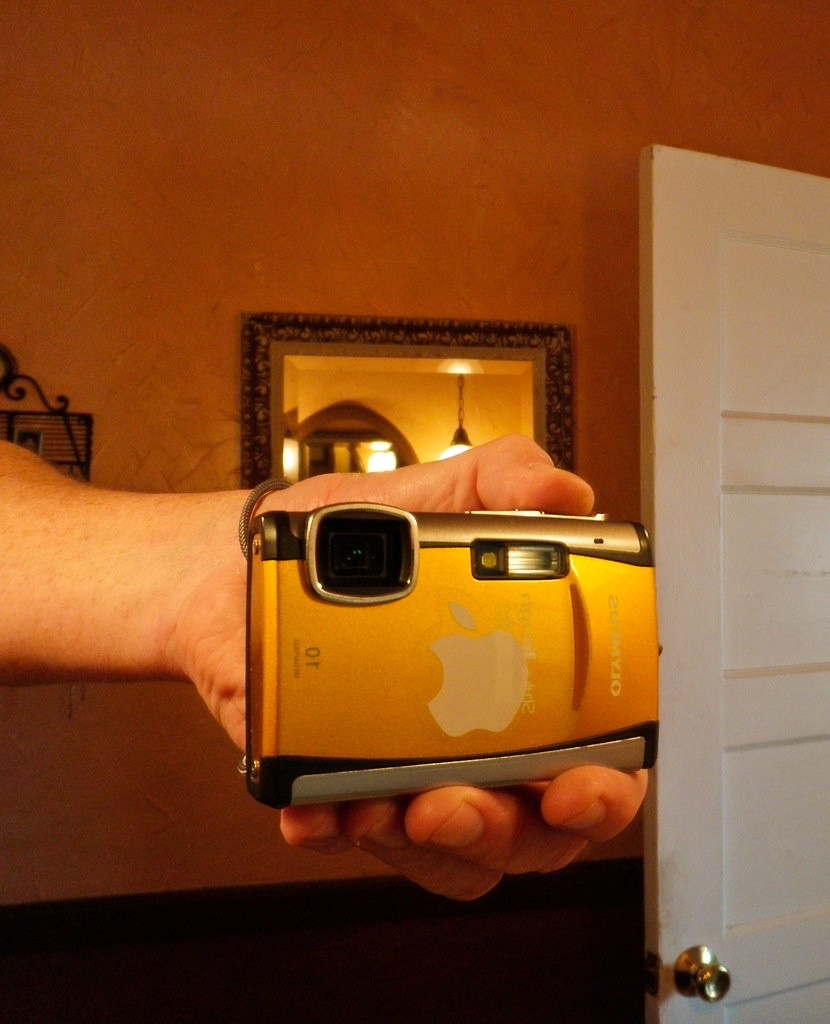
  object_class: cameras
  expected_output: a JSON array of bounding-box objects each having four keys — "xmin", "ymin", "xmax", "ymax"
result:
[{"xmin": 243, "ymin": 501, "xmax": 659, "ymax": 809}]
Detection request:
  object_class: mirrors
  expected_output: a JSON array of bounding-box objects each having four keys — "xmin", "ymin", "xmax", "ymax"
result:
[{"xmin": 237, "ymin": 314, "xmax": 577, "ymax": 523}]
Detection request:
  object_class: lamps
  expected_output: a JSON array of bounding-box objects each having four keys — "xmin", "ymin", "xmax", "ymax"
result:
[{"xmin": 435, "ymin": 375, "xmax": 474, "ymax": 460}]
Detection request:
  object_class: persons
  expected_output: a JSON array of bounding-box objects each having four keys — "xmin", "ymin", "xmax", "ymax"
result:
[{"xmin": 0, "ymin": 433, "xmax": 649, "ymax": 898}]
